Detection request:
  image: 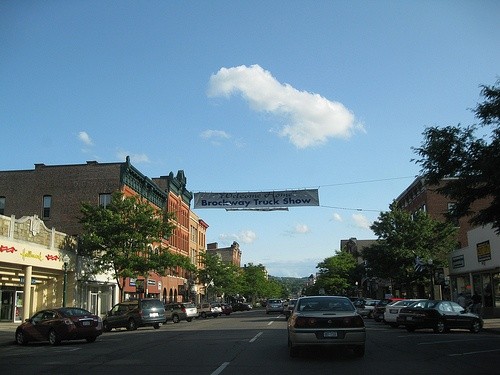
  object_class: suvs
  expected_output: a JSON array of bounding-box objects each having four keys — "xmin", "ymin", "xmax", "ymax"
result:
[{"xmin": 102, "ymin": 297, "xmax": 166, "ymax": 331}]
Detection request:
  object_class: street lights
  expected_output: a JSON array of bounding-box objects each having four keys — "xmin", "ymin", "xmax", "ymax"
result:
[
  {"xmin": 204, "ymin": 282, "xmax": 208, "ymax": 303},
  {"xmin": 428, "ymin": 257, "xmax": 435, "ymax": 300},
  {"xmin": 63, "ymin": 254, "xmax": 70, "ymax": 307},
  {"xmin": 355, "ymin": 281, "xmax": 359, "ymax": 297}
]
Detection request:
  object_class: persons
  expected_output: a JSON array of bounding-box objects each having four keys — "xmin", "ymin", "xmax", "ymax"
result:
[{"xmin": 457, "ymin": 293, "xmax": 465, "ymax": 307}]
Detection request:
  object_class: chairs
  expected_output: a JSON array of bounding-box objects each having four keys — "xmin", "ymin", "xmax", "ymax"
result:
[
  {"xmin": 302, "ymin": 305, "xmax": 312, "ymax": 312},
  {"xmin": 332, "ymin": 304, "xmax": 345, "ymax": 311}
]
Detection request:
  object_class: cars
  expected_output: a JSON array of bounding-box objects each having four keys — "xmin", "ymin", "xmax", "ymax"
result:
[
  {"xmin": 265, "ymin": 299, "xmax": 308, "ymax": 321},
  {"xmin": 372, "ymin": 298, "xmax": 408, "ymax": 323},
  {"xmin": 286, "ymin": 295, "xmax": 367, "ymax": 357},
  {"xmin": 384, "ymin": 299, "xmax": 428, "ymax": 328},
  {"xmin": 334, "ymin": 296, "xmax": 381, "ymax": 320},
  {"xmin": 162, "ymin": 302, "xmax": 253, "ymax": 324},
  {"xmin": 15, "ymin": 307, "xmax": 104, "ymax": 346},
  {"xmin": 396, "ymin": 298, "xmax": 484, "ymax": 335}
]
